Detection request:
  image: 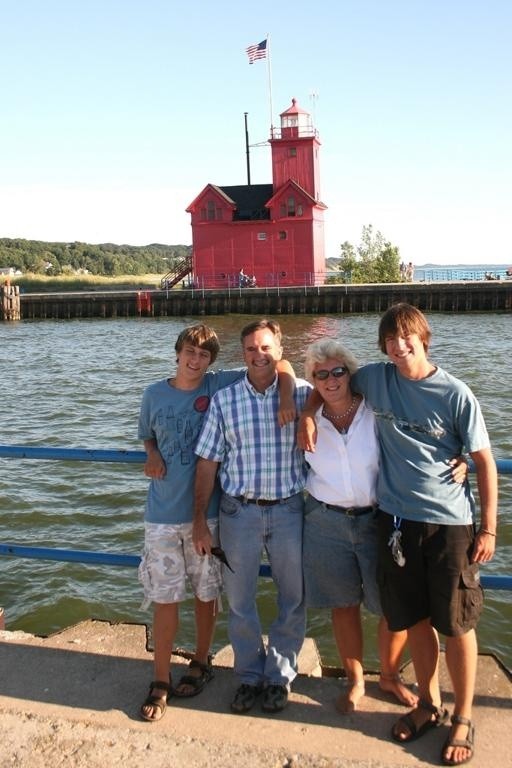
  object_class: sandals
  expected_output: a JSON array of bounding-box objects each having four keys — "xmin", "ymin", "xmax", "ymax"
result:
[
  {"xmin": 440, "ymin": 717, "xmax": 478, "ymax": 766},
  {"xmin": 173, "ymin": 655, "xmax": 219, "ymax": 700},
  {"xmin": 388, "ymin": 699, "xmax": 450, "ymax": 743},
  {"xmin": 138, "ymin": 671, "xmax": 172, "ymax": 724}
]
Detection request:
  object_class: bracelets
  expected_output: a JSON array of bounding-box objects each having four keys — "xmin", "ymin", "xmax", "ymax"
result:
[{"xmin": 477, "ymin": 526, "xmax": 501, "ymax": 539}]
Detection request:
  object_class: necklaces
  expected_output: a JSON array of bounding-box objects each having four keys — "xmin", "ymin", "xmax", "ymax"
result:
[{"xmin": 320, "ymin": 394, "xmax": 358, "ymax": 419}]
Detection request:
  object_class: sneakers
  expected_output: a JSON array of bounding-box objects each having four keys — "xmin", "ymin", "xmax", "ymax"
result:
[
  {"xmin": 229, "ymin": 679, "xmax": 260, "ymax": 715},
  {"xmin": 261, "ymin": 680, "xmax": 292, "ymax": 716}
]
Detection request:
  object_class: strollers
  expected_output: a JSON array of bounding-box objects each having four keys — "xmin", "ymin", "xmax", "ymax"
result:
[{"xmin": 240, "ymin": 275, "xmax": 258, "ymax": 288}]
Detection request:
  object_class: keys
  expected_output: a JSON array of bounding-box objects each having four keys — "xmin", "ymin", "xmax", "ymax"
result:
[{"xmin": 389, "ymin": 527, "xmax": 406, "ymax": 569}]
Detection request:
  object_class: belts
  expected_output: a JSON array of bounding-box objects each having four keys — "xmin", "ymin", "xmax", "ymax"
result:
[
  {"xmin": 230, "ymin": 491, "xmax": 297, "ymax": 508},
  {"xmin": 310, "ymin": 497, "xmax": 380, "ymax": 519}
]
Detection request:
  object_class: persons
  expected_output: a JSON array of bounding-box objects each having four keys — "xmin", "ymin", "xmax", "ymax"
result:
[
  {"xmin": 133, "ymin": 323, "xmax": 296, "ymax": 722},
  {"xmin": 238, "ymin": 268, "xmax": 246, "ymax": 288},
  {"xmin": 397, "ymin": 261, "xmax": 416, "ymax": 282},
  {"xmin": 303, "ymin": 337, "xmax": 471, "ymax": 715},
  {"xmin": 191, "ymin": 318, "xmax": 315, "ymax": 714},
  {"xmin": 482, "ymin": 264, "xmax": 512, "ymax": 281},
  {"xmin": 295, "ymin": 302, "xmax": 497, "ymax": 767}
]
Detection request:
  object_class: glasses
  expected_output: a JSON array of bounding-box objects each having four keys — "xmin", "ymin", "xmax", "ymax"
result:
[{"xmin": 310, "ymin": 364, "xmax": 350, "ymax": 384}]
